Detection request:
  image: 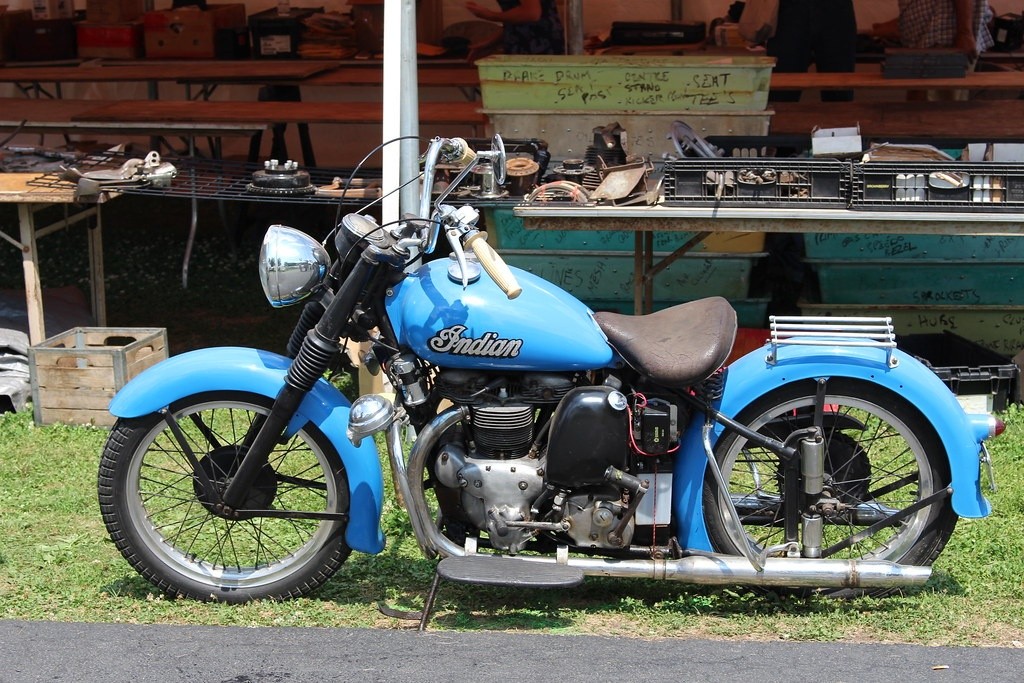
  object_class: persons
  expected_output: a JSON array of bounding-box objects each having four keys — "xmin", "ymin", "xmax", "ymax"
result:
[
  {"xmin": 872, "ymin": 0, "xmax": 995, "ymax": 102},
  {"xmin": 737, "ymin": 0, "xmax": 901, "ymax": 201},
  {"xmin": 465, "ymin": 0, "xmax": 566, "ymax": 71}
]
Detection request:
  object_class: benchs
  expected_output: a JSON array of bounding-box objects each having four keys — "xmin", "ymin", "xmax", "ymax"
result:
[
  {"xmin": 0, "ymin": 94, "xmax": 490, "ymax": 166},
  {"xmin": 766, "ymin": 100, "xmax": 1024, "ymax": 150}
]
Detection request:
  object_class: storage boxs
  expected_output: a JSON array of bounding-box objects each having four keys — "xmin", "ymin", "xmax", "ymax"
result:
[
  {"xmin": 475, "ymin": 54, "xmax": 776, "ymax": 110},
  {"xmin": 988, "ymin": 12, "xmax": 1024, "ymax": 51},
  {"xmin": 490, "ymin": 246, "xmax": 770, "ymax": 301},
  {"xmin": 895, "ymin": 327, "xmax": 1020, "ymax": 415},
  {"xmin": 85, "ymin": 0, "xmax": 156, "ymax": 24},
  {"xmin": 478, "ymin": 106, "xmax": 773, "ymax": 167},
  {"xmin": 578, "ymin": 296, "xmax": 770, "ymax": 328},
  {"xmin": 481, "ymin": 206, "xmax": 768, "ymax": 256},
  {"xmin": 31, "ymin": 0, "xmax": 75, "ymax": 19},
  {"xmin": 721, "ymin": 327, "xmax": 769, "ymax": 366},
  {"xmin": 798, "ymin": 300, "xmax": 1024, "ymax": 360},
  {"xmin": 801, "ymin": 224, "xmax": 1024, "ymax": 265},
  {"xmin": 27, "ymin": 325, "xmax": 168, "ymax": 430},
  {"xmin": 76, "ymin": 20, "xmax": 140, "ymax": 59},
  {"xmin": 794, "ymin": 254, "xmax": 1024, "ymax": 310},
  {"xmin": 144, "ymin": 3, "xmax": 247, "ymax": 57},
  {"xmin": 249, "ymin": 6, "xmax": 325, "ymax": 56}
]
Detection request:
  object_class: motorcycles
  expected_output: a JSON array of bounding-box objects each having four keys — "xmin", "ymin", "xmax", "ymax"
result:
[{"xmin": 91, "ymin": 131, "xmax": 1006, "ymax": 606}]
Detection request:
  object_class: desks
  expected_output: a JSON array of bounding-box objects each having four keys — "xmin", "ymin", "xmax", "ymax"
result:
[
  {"xmin": 303, "ymin": 67, "xmax": 482, "ymax": 103},
  {"xmin": 512, "ymin": 186, "xmax": 1023, "ymax": 316},
  {"xmin": 1, "ymin": 172, "xmax": 126, "ymax": 350},
  {"xmin": 769, "ymin": 70, "xmax": 1024, "ymax": 89},
  {"xmin": 0, "ymin": 58, "xmax": 342, "ymax": 156}
]
